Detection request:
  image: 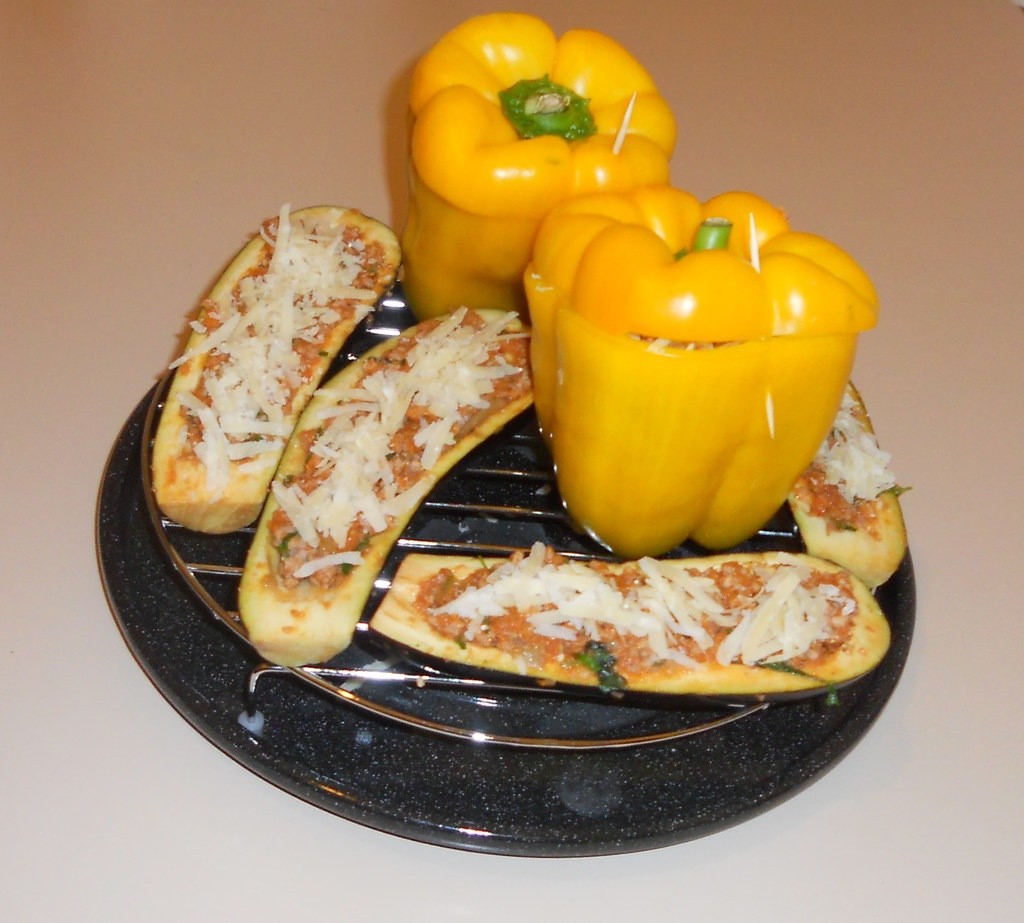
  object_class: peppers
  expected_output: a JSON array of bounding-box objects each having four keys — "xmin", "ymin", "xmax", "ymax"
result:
[{"xmin": 399, "ymin": 11, "xmax": 882, "ymax": 559}]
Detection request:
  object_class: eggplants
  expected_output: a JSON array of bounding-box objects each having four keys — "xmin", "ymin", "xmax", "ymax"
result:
[{"xmin": 146, "ymin": 203, "xmax": 910, "ymax": 710}]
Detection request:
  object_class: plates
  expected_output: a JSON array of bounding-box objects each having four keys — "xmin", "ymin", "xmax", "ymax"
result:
[{"xmin": 86, "ymin": 253, "xmax": 916, "ymax": 854}]
